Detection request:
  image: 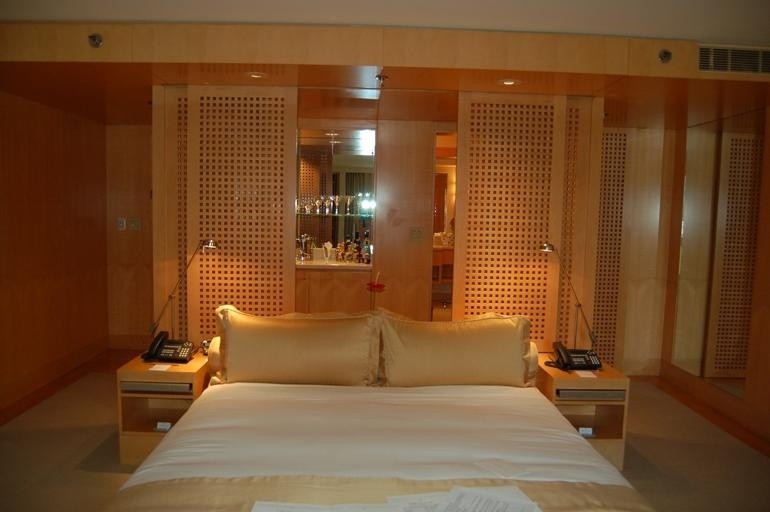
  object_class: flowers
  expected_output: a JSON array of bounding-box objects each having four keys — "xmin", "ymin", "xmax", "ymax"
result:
[{"xmin": 366, "ymin": 271, "xmax": 385, "ymax": 311}]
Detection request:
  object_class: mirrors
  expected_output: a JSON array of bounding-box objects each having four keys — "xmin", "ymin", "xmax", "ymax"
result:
[
  {"xmin": 297, "ymin": 89, "xmax": 377, "ymax": 261},
  {"xmin": 670, "ymin": 112, "xmax": 767, "ymax": 404}
]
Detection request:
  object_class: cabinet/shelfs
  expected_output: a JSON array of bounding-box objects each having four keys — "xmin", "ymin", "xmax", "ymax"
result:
[{"xmin": 295, "ymin": 88, "xmax": 378, "ymax": 313}]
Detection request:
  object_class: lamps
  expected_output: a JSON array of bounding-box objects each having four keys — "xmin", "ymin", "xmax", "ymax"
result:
[
  {"xmin": 149, "ymin": 239, "xmax": 219, "ymax": 335},
  {"xmin": 537, "ymin": 241, "xmax": 595, "ymax": 342}
]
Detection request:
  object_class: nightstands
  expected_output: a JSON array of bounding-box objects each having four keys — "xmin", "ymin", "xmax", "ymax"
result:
[
  {"xmin": 534, "ymin": 352, "xmax": 630, "ymax": 472},
  {"xmin": 116, "ymin": 347, "xmax": 210, "ymax": 463}
]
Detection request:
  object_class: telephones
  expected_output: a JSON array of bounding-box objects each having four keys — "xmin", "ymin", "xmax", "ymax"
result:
[
  {"xmin": 149, "ymin": 331, "xmax": 193, "ymax": 361},
  {"xmin": 553, "ymin": 342, "xmax": 601, "ymax": 369}
]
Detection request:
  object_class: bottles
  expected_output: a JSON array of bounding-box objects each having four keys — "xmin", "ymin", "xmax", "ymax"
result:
[{"xmin": 334, "ymin": 230, "xmax": 371, "ymax": 265}]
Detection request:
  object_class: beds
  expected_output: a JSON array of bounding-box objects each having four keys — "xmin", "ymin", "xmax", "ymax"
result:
[{"xmin": 106, "ymin": 375, "xmax": 654, "ymax": 512}]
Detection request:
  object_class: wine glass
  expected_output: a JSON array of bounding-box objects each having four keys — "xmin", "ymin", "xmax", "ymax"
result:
[
  {"xmin": 322, "ymin": 244, "xmax": 332, "ymax": 264},
  {"xmin": 299, "ymin": 192, "xmax": 374, "ymax": 215}
]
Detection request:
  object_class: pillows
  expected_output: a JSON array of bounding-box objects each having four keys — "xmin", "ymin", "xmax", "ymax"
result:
[
  {"xmin": 210, "ymin": 304, "xmax": 381, "ymax": 385},
  {"xmin": 381, "ymin": 310, "xmax": 539, "ymax": 387}
]
